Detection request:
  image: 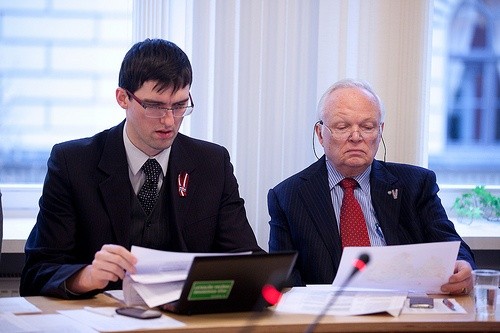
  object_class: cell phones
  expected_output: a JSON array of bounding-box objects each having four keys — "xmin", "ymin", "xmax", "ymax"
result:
[
  {"xmin": 115, "ymin": 305, "xmax": 162, "ymax": 320},
  {"xmin": 409, "ymin": 296, "xmax": 434, "ymax": 309}
]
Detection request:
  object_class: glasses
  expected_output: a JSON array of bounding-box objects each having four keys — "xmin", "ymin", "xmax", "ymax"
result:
[
  {"xmin": 323, "ymin": 122, "xmax": 382, "ymax": 141},
  {"xmin": 122, "ymin": 88, "xmax": 194, "ymax": 119}
]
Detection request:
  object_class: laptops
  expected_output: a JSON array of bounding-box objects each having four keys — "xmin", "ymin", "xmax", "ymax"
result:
[{"xmin": 158, "ymin": 249, "xmax": 299, "ymax": 313}]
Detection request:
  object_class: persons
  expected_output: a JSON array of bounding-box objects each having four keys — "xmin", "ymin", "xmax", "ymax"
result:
[
  {"xmin": 19, "ymin": 38, "xmax": 259, "ymax": 299},
  {"xmin": 267, "ymin": 80, "xmax": 477, "ymax": 297}
]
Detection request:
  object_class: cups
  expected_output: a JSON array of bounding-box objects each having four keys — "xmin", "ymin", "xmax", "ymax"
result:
[{"xmin": 470, "ymin": 269, "xmax": 500, "ymax": 316}]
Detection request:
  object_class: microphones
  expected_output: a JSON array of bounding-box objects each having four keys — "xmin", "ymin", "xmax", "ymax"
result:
[{"xmin": 307, "ymin": 253, "xmax": 371, "ymax": 332}]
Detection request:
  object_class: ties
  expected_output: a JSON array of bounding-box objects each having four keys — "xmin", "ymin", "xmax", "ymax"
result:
[
  {"xmin": 340, "ymin": 178, "xmax": 371, "ymax": 253},
  {"xmin": 136, "ymin": 159, "xmax": 162, "ymax": 218}
]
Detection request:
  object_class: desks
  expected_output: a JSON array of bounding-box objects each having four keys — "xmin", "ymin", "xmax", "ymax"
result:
[{"xmin": 0, "ymin": 280, "xmax": 500, "ymax": 333}]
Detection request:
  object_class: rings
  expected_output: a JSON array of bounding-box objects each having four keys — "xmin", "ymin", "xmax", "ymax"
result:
[{"xmin": 461, "ymin": 288, "xmax": 467, "ymax": 294}]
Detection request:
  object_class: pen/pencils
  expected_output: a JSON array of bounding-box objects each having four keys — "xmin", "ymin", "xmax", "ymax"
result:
[
  {"xmin": 443, "ymin": 298, "xmax": 456, "ymax": 311},
  {"xmin": 84, "ymin": 307, "xmax": 113, "ymax": 317}
]
